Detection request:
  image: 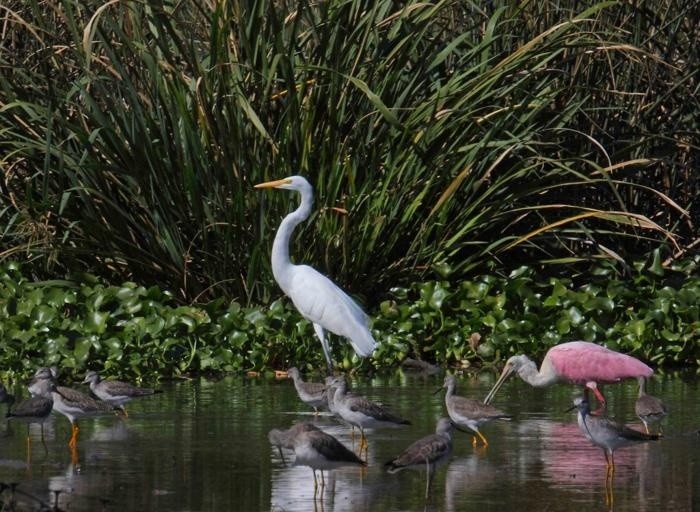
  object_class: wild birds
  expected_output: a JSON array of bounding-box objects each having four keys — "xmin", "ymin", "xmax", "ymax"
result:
[
  {"xmin": 567, "ymin": 395, "xmax": 662, "ymax": 470},
  {"xmin": 4, "ymin": 367, "xmax": 51, "ymax": 446},
  {"xmin": 320, "ymin": 376, "xmax": 412, "ymax": 448},
  {"xmin": 0, "ymin": 383, "xmax": 13, "ymax": 406},
  {"xmin": 382, "ymin": 416, "xmax": 456, "ymax": 495},
  {"xmin": 250, "ymin": 174, "xmax": 380, "ymax": 371},
  {"xmin": 480, "ymin": 340, "xmax": 652, "ymax": 414},
  {"xmin": 284, "ymin": 365, "xmax": 326, "ymax": 416},
  {"xmin": 629, "ymin": 371, "xmax": 669, "ymax": 436},
  {"xmin": 267, "ymin": 420, "xmax": 368, "ymax": 485},
  {"xmin": 433, "ymin": 374, "xmax": 509, "ymax": 448},
  {"xmin": 80, "ymin": 371, "xmax": 165, "ymax": 417},
  {"xmin": 29, "ymin": 365, "xmax": 124, "ymax": 438}
]
